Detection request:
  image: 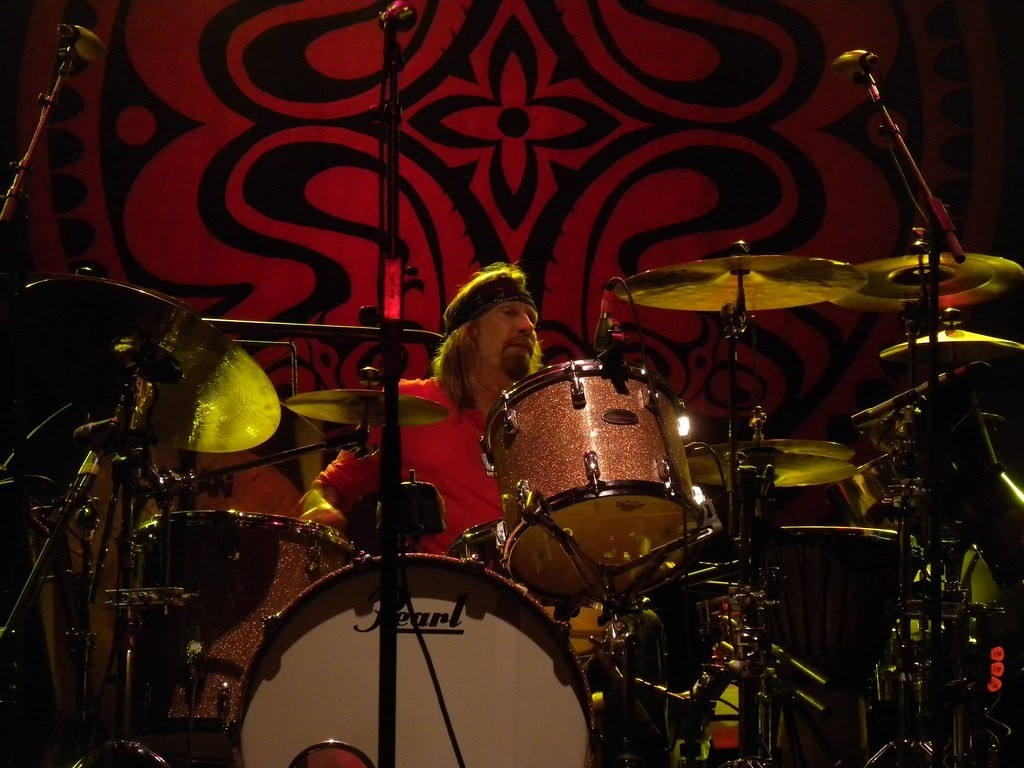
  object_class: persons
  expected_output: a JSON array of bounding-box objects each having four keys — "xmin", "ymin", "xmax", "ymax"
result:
[{"xmin": 297, "ymin": 263, "xmax": 541, "ymax": 558}]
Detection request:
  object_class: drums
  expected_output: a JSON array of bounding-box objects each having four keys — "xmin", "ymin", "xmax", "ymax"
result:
[
  {"xmin": 240, "ymin": 553, "xmax": 603, "ymax": 768},
  {"xmin": 119, "ymin": 511, "xmax": 367, "ymax": 726},
  {"xmin": 479, "ymin": 361, "xmax": 709, "ymax": 597}
]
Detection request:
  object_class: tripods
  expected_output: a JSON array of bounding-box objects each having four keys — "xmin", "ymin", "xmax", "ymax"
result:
[
  {"xmin": 662, "ymin": 278, "xmax": 1023, "ymax": 768},
  {"xmin": 38, "ymin": 399, "xmax": 171, "ymax": 768}
]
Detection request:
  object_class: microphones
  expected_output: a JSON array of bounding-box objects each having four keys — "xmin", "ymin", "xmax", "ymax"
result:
[
  {"xmin": 57, "ymin": 23, "xmax": 108, "ymax": 62},
  {"xmin": 831, "ymin": 49, "xmax": 879, "ymax": 74},
  {"xmin": 851, "ymin": 365, "xmax": 972, "ymax": 429},
  {"xmin": 594, "ymin": 282, "xmax": 618, "ymax": 352},
  {"xmin": 378, "ymin": 7, "xmax": 417, "ymax": 33},
  {"xmin": 76, "ymin": 416, "xmax": 121, "ymax": 452}
]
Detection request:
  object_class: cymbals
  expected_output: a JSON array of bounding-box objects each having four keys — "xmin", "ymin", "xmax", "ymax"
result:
[
  {"xmin": 0, "ymin": 272, "xmax": 281, "ymax": 453},
  {"xmin": 683, "ymin": 438, "xmax": 861, "ymax": 488},
  {"xmin": 880, "ymin": 328, "xmax": 1024, "ymax": 359},
  {"xmin": 617, "ymin": 253, "xmax": 868, "ymax": 312},
  {"xmin": 832, "ymin": 253, "xmax": 1024, "ymax": 315},
  {"xmin": 285, "ymin": 389, "xmax": 452, "ymax": 427}
]
